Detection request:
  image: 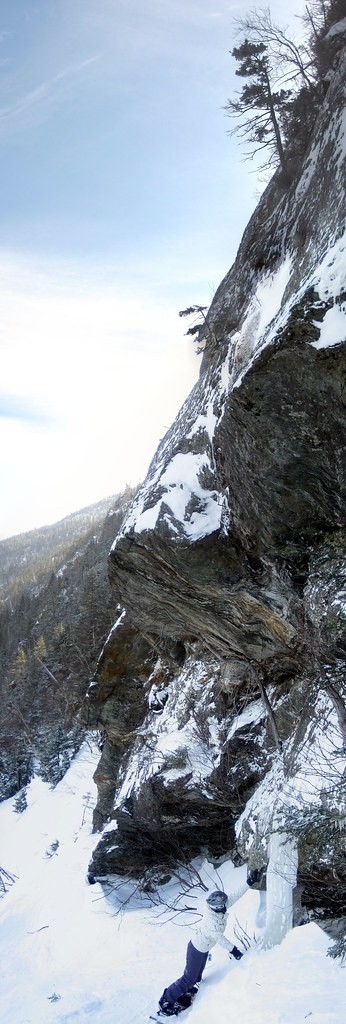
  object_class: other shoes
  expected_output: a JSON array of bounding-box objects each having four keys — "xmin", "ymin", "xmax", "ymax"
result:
[
  {"xmin": 175, "ymin": 987, "xmax": 199, "ymax": 1010},
  {"xmin": 157, "ymin": 988, "xmax": 179, "ymax": 1017}
]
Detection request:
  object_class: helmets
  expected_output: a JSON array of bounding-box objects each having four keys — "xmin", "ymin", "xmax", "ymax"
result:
[{"xmin": 206, "ymin": 890, "xmax": 228, "ymax": 906}]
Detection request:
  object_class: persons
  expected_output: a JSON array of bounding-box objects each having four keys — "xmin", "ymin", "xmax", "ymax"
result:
[{"xmin": 159, "ymin": 891, "xmax": 244, "ymax": 1015}]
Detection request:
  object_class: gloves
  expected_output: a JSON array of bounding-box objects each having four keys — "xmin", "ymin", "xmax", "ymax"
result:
[
  {"xmin": 246, "ymin": 868, "xmax": 260, "ymax": 885},
  {"xmin": 230, "ymin": 945, "xmax": 242, "ymax": 960}
]
